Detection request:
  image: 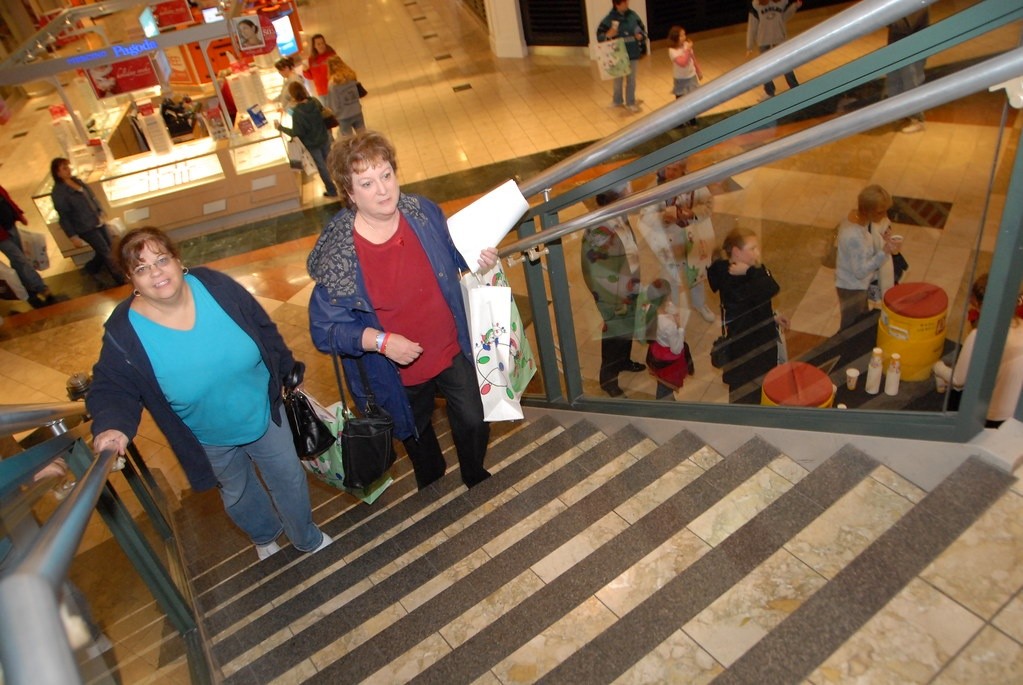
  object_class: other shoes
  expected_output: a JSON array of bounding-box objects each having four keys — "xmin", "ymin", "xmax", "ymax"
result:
[
  {"xmin": 624, "ymin": 104, "xmax": 638, "ymax": 111},
  {"xmin": 324, "ymin": 193, "xmax": 335, "ymax": 197},
  {"xmin": 314, "ymin": 532, "xmax": 332, "ymax": 552},
  {"xmin": 698, "ymin": 305, "xmax": 716, "ymax": 322},
  {"xmin": 255, "ymin": 542, "xmax": 279, "ymax": 560},
  {"xmin": 628, "ymin": 361, "xmax": 645, "ymax": 373},
  {"xmin": 603, "ymin": 385, "xmax": 623, "ymax": 396},
  {"xmin": 903, "ymin": 118, "xmax": 924, "ymax": 132}
]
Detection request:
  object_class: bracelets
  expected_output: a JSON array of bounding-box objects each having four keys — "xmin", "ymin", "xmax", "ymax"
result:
[{"xmin": 376, "ymin": 331, "xmax": 391, "ymax": 353}]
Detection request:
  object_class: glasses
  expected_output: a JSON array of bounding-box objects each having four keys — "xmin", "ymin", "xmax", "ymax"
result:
[{"xmin": 128, "ymin": 254, "xmax": 174, "ymax": 278}]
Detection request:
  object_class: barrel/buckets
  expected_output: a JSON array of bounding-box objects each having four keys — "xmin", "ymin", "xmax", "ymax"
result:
[
  {"xmin": 876, "ymin": 282, "xmax": 949, "ymax": 382},
  {"xmin": 762, "ymin": 362, "xmax": 836, "ymax": 412}
]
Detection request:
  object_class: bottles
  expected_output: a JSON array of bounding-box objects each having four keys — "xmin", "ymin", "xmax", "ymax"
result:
[
  {"xmin": 885, "ymin": 352, "xmax": 901, "ymax": 394},
  {"xmin": 866, "ymin": 348, "xmax": 882, "ymax": 394},
  {"xmin": 935, "ymin": 372, "xmax": 946, "ymax": 393}
]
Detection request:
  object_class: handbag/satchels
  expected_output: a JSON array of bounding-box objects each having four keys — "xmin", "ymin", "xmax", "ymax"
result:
[
  {"xmin": 460, "ymin": 260, "xmax": 537, "ymax": 421},
  {"xmin": 594, "ymin": 35, "xmax": 631, "ymax": 81},
  {"xmin": 0, "ymin": 261, "xmax": 28, "ymax": 302},
  {"xmin": 711, "ymin": 337, "xmax": 729, "ymax": 367},
  {"xmin": 321, "ymin": 105, "xmax": 339, "ymax": 128},
  {"xmin": 342, "ymin": 398, "xmax": 397, "ymax": 488},
  {"xmin": 355, "ymin": 78, "xmax": 367, "ymax": 98},
  {"xmin": 19, "ymin": 223, "xmax": 50, "ymax": 271},
  {"xmin": 284, "ymin": 365, "xmax": 336, "ymax": 459},
  {"xmin": 681, "ymin": 209, "xmax": 716, "ymax": 269},
  {"xmin": 820, "ymin": 220, "xmax": 843, "ymax": 268},
  {"xmin": 295, "ymin": 386, "xmax": 393, "ymax": 504}
]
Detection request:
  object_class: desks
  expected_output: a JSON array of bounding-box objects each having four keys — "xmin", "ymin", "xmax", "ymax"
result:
[{"xmin": 751, "ymin": 308, "xmax": 963, "ymax": 412}]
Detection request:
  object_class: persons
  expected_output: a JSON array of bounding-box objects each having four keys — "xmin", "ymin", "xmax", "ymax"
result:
[
  {"xmin": 267, "ymin": 58, "xmax": 305, "ymax": 117},
  {"xmin": 667, "ymin": 26, "xmax": 702, "ymax": 131},
  {"xmin": 638, "ymin": 158, "xmax": 715, "ymax": 322},
  {"xmin": 580, "ymin": 183, "xmax": 646, "ymax": 398},
  {"xmin": 303, "ymin": 34, "xmax": 335, "ymax": 109},
  {"xmin": 886, "ymin": 7, "xmax": 931, "ymax": 132},
  {"xmin": 707, "ymin": 228, "xmax": 789, "ymax": 405},
  {"xmin": 274, "ymin": 81, "xmax": 337, "ymax": 195},
  {"xmin": 87, "ymin": 227, "xmax": 331, "ymax": 560},
  {"xmin": 746, "ymin": 0, "xmax": 801, "ymax": 96},
  {"xmin": 326, "ymin": 56, "xmax": 365, "ymax": 134},
  {"xmin": 0, "ymin": 187, "xmax": 56, "ymax": 304},
  {"xmin": 837, "ymin": 185, "xmax": 901, "ymax": 330},
  {"xmin": 50, "ymin": 158, "xmax": 125, "ymax": 284},
  {"xmin": 307, "ymin": 133, "xmax": 498, "ymax": 490},
  {"xmin": 596, "ymin": 0, "xmax": 647, "ymax": 112},
  {"xmin": 238, "ymin": 20, "xmax": 262, "ymax": 45},
  {"xmin": 933, "ymin": 275, "xmax": 1023, "ymax": 427},
  {"xmin": 645, "ymin": 279, "xmax": 685, "ymax": 401}
]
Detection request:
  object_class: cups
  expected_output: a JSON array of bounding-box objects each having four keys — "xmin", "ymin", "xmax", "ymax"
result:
[
  {"xmin": 846, "ymin": 368, "xmax": 859, "ymax": 389},
  {"xmin": 890, "ymin": 235, "xmax": 903, "ymax": 255}
]
